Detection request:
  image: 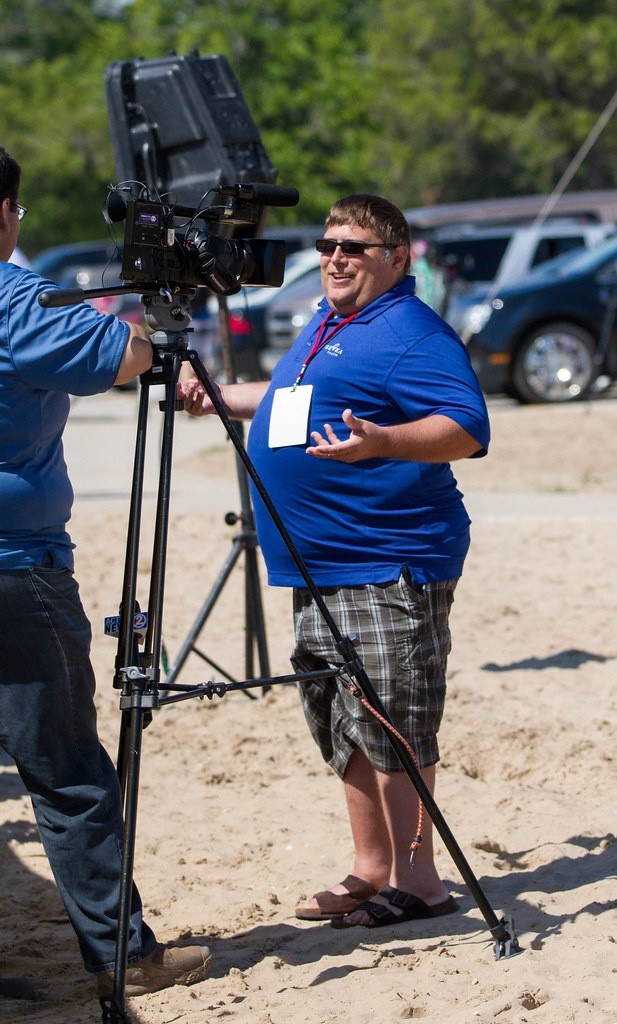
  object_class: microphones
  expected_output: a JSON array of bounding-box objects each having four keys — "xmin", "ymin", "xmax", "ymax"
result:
[{"xmin": 216, "ymin": 183, "xmax": 300, "ymax": 207}]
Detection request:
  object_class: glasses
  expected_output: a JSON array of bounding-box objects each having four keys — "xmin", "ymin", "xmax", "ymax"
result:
[
  {"xmin": 10, "ymin": 203, "xmax": 28, "ymax": 221},
  {"xmin": 315, "ymin": 239, "xmax": 396, "ymax": 256}
]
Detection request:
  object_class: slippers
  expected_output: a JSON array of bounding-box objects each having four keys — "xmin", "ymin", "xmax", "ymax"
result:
[
  {"xmin": 295, "ymin": 874, "xmax": 379, "ymax": 919},
  {"xmin": 331, "ymin": 884, "xmax": 459, "ymax": 929}
]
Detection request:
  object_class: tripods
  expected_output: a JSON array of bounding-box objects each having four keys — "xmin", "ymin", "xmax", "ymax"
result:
[{"xmin": 37, "ymin": 282, "xmax": 530, "ymax": 1024}]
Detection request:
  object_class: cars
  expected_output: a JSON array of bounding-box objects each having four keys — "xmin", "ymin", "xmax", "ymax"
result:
[{"xmin": 29, "ymin": 189, "xmax": 617, "ymax": 403}]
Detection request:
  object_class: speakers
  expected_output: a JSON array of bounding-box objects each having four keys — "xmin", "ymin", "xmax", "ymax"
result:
[{"xmin": 106, "ymin": 50, "xmax": 278, "ymax": 236}]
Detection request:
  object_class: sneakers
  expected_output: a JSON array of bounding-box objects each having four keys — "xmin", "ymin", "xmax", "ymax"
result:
[{"xmin": 96, "ymin": 943, "xmax": 213, "ymax": 999}]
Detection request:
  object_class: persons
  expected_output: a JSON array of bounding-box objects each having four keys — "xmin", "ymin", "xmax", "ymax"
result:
[
  {"xmin": 0, "ymin": 149, "xmax": 214, "ymax": 1001},
  {"xmin": 176, "ymin": 194, "xmax": 490, "ymax": 929}
]
